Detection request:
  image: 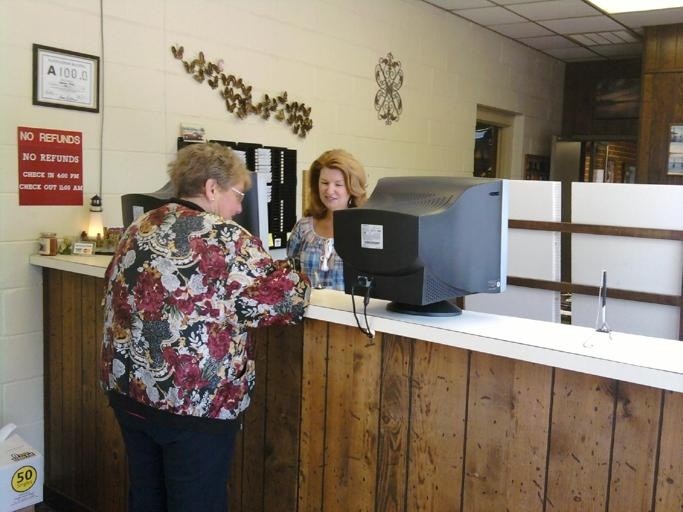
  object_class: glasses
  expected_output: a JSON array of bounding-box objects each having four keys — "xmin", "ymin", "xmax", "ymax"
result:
[{"xmin": 231, "ymin": 187, "xmax": 245, "ymax": 202}]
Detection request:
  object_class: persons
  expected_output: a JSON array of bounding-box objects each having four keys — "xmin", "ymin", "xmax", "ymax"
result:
[
  {"xmin": 99, "ymin": 141, "xmax": 313, "ymax": 511},
  {"xmin": 286, "ymin": 149, "xmax": 368, "ymax": 291}
]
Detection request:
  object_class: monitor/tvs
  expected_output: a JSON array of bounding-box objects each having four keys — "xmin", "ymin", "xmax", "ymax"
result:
[{"xmin": 332, "ymin": 174, "xmax": 509, "ymax": 318}]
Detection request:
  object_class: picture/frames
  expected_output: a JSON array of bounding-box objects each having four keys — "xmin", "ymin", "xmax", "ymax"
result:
[{"xmin": 32, "ymin": 44, "xmax": 99, "ymax": 113}]
